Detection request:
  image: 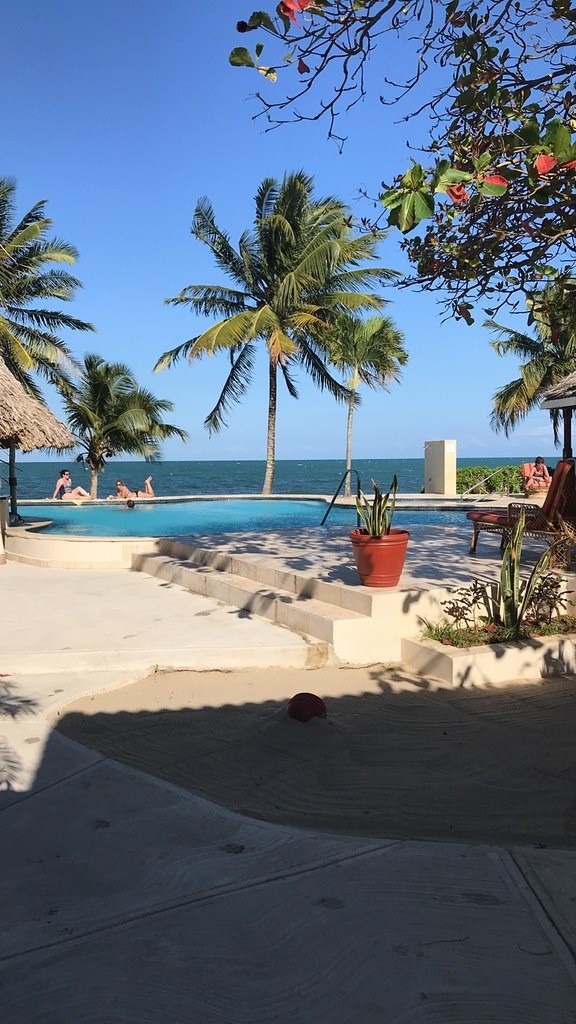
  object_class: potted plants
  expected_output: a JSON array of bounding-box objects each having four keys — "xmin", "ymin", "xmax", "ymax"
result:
[{"xmin": 351, "ymin": 473, "xmax": 411, "ymax": 588}]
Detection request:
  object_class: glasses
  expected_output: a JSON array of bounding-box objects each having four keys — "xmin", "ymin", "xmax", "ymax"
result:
[
  {"xmin": 65, "ymin": 474, "xmax": 69, "ymax": 476},
  {"xmin": 116, "ymin": 484, "xmax": 121, "ymax": 486}
]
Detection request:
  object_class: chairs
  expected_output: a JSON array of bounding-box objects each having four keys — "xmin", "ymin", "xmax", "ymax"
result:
[
  {"xmin": 467, "ymin": 456, "xmax": 576, "ymax": 564},
  {"xmin": 522, "ymin": 463, "xmax": 552, "ymax": 498}
]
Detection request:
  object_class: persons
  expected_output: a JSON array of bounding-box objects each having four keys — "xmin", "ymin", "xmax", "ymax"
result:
[
  {"xmin": 126, "ymin": 500, "xmax": 135, "ymax": 509},
  {"xmin": 52, "ymin": 470, "xmax": 95, "ymax": 501},
  {"xmin": 109, "ymin": 476, "xmax": 155, "ymax": 500},
  {"xmin": 522, "ymin": 457, "xmax": 550, "ymax": 491}
]
